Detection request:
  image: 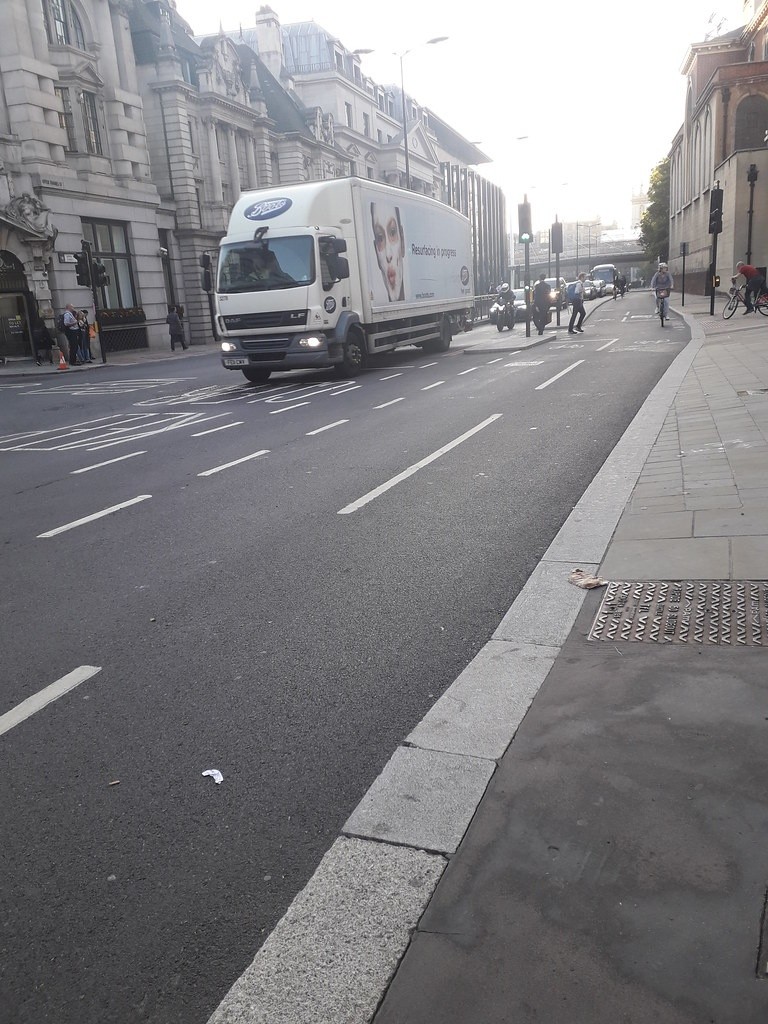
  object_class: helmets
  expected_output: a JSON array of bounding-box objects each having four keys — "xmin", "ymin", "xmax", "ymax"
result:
[
  {"xmin": 657, "ymin": 263, "xmax": 669, "ymax": 272},
  {"xmin": 500, "ymin": 283, "xmax": 510, "ymax": 293}
]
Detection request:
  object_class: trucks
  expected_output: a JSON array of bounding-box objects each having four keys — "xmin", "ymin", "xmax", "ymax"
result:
[{"xmin": 198, "ymin": 175, "xmax": 476, "ymax": 384}]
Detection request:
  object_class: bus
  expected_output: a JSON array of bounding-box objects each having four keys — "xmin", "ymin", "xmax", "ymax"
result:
[{"xmin": 590, "ymin": 264, "xmax": 619, "ymax": 295}]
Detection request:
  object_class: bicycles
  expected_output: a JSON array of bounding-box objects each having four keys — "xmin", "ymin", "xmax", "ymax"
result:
[
  {"xmin": 722, "ymin": 278, "xmax": 768, "ymax": 319},
  {"xmin": 651, "ymin": 286, "xmax": 673, "ymax": 326}
]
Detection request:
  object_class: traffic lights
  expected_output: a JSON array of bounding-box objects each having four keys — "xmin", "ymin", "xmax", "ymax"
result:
[
  {"xmin": 92, "ymin": 261, "xmax": 110, "ymax": 287},
  {"xmin": 73, "ymin": 251, "xmax": 93, "ymax": 286}
]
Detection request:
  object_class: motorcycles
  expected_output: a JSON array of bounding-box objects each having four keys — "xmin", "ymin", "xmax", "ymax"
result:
[{"xmin": 494, "ymin": 299, "xmax": 518, "ymax": 333}]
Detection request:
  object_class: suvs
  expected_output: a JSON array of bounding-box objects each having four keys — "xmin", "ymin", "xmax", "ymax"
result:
[{"xmin": 532, "ymin": 277, "xmax": 569, "ymax": 310}]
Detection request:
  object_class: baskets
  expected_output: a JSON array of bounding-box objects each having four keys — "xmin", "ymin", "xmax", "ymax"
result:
[{"xmin": 655, "ymin": 288, "xmax": 670, "ymax": 298}]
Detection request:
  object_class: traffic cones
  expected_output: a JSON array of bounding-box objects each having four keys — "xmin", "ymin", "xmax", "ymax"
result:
[{"xmin": 57, "ymin": 352, "xmax": 70, "ymax": 370}]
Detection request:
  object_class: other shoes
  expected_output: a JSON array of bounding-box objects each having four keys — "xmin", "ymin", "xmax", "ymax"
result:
[
  {"xmin": 568, "ymin": 329, "xmax": 577, "ymax": 334},
  {"xmin": 171, "ymin": 349, "xmax": 176, "ymax": 352},
  {"xmin": 576, "ymin": 325, "xmax": 583, "ymax": 332},
  {"xmin": 67, "ymin": 354, "xmax": 98, "ymax": 367},
  {"xmin": 537, "ymin": 331, "xmax": 543, "ymax": 335},
  {"xmin": 183, "ymin": 346, "xmax": 189, "ymax": 350}
]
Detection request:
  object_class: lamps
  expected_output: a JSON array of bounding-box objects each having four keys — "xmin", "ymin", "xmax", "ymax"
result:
[{"xmin": 157, "ymin": 247, "xmax": 167, "ymax": 258}]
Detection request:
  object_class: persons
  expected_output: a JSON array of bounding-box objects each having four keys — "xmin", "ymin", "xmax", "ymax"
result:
[
  {"xmin": 650, "ymin": 263, "xmax": 674, "ymax": 320},
  {"xmin": 34, "ymin": 309, "xmax": 56, "ymax": 366},
  {"xmin": 166, "ymin": 307, "xmax": 189, "ymax": 352},
  {"xmin": 64, "ymin": 303, "xmax": 96, "ymax": 365},
  {"xmin": 495, "ymin": 282, "xmax": 515, "ymax": 319},
  {"xmin": 533, "ymin": 273, "xmax": 551, "ymax": 335},
  {"xmin": 613, "ymin": 275, "xmax": 626, "ymax": 299},
  {"xmin": 248, "ymin": 256, "xmax": 271, "ymax": 281},
  {"xmin": 370, "ymin": 201, "xmax": 406, "ymax": 302},
  {"xmin": 568, "ymin": 272, "xmax": 588, "ymax": 334},
  {"xmin": 731, "ymin": 261, "xmax": 768, "ymax": 315}
]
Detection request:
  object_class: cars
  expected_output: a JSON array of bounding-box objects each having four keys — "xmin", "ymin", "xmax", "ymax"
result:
[
  {"xmin": 488, "ymin": 288, "xmax": 535, "ymax": 324},
  {"xmin": 564, "ymin": 280, "xmax": 607, "ymax": 303}
]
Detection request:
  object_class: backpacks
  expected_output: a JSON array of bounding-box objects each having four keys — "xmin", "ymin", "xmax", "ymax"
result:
[
  {"xmin": 54, "ymin": 314, "xmax": 66, "ymax": 333},
  {"xmin": 567, "ymin": 283, "xmax": 582, "ymax": 302}
]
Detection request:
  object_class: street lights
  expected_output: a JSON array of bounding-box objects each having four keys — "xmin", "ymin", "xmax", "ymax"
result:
[
  {"xmin": 578, "ymin": 222, "xmax": 603, "ymax": 271},
  {"xmin": 352, "ymin": 34, "xmax": 451, "ymax": 193},
  {"xmin": 586, "ymin": 232, "xmax": 607, "ymax": 255}
]
